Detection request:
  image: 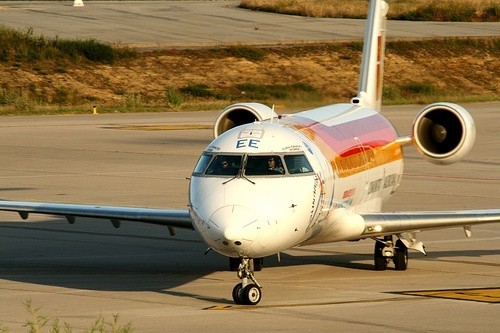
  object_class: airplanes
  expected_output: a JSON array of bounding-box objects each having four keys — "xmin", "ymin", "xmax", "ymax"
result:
[{"xmin": 0, "ymin": 0, "xmax": 499, "ymax": 306}]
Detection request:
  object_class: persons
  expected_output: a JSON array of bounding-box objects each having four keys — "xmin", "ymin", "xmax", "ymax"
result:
[
  {"xmin": 218, "ymin": 159, "xmax": 228, "ymax": 168},
  {"xmin": 259, "ymin": 158, "xmax": 282, "ymax": 172}
]
